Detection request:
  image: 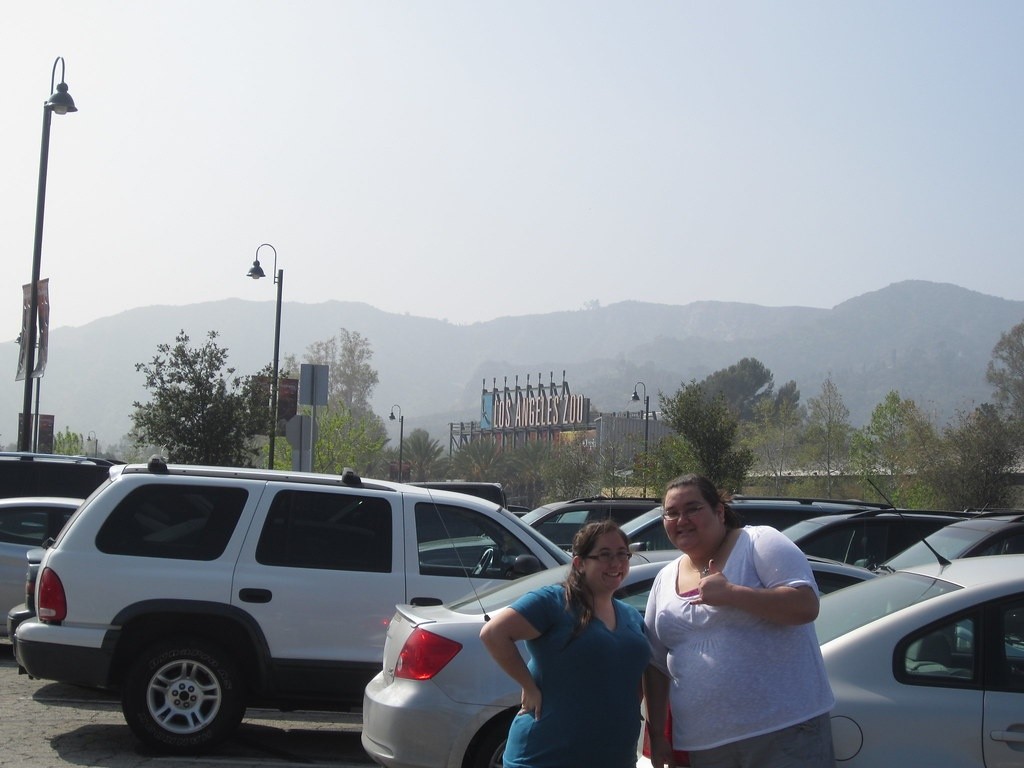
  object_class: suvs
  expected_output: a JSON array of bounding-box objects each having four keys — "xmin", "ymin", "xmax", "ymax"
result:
[
  {"xmin": 1, "ymin": 447, "xmax": 129, "ymax": 537},
  {"xmin": 13, "ymin": 456, "xmax": 576, "ymax": 755}
]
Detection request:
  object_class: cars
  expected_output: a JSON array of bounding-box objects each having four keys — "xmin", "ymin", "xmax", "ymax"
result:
[
  {"xmin": 362, "ymin": 494, "xmax": 1022, "ymax": 767},
  {"xmin": 0, "ymin": 497, "xmax": 85, "ymax": 646}
]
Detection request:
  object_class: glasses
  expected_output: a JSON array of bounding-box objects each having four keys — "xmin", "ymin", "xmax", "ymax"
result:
[
  {"xmin": 661, "ymin": 502, "xmax": 712, "ymax": 521},
  {"xmin": 586, "ymin": 549, "xmax": 632, "ymax": 562}
]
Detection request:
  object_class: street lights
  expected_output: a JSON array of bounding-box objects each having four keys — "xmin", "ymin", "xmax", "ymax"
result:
[
  {"xmin": 248, "ymin": 243, "xmax": 283, "ymax": 470},
  {"xmin": 632, "ymin": 381, "xmax": 649, "ymax": 499},
  {"xmin": 389, "ymin": 404, "xmax": 404, "ymax": 484},
  {"xmin": 19, "ymin": 56, "xmax": 81, "ymax": 460}
]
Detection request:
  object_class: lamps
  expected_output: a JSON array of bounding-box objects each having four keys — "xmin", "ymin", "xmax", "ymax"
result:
[
  {"xmin": 43, "ymin": 82, "xmax": 79, "ymax": 115},
  {"xmin": 246, "ymin": 260, "xmax": 266, "ymax": 279}
]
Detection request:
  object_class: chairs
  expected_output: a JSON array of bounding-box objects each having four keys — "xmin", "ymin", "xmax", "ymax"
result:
[{"xmin": 905, "ymin": 633, "xmax": 954, "ymax": 676}]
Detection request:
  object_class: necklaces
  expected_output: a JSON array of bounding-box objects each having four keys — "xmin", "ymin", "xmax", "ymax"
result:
[{"xmin": 689, "ymin": 537, "xmax": 725, "ymax": 575}]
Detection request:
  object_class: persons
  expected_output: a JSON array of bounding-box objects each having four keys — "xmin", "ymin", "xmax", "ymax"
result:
[
  {"xmin": 479, "ymin": 520, "xmax": 649, "ymax": 767},
  {"xmin": 640, "ymin": 475, "xmax": 843, "ymax": 768}
]
